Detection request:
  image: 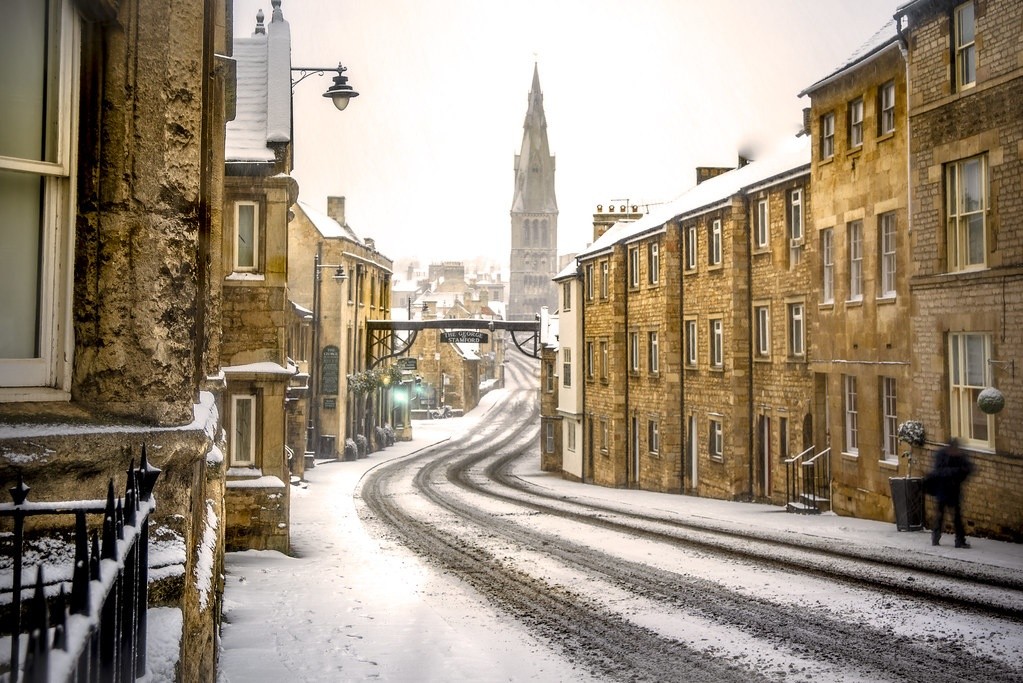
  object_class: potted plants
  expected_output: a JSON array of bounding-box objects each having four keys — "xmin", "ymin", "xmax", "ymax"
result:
[{"xmin": 888, "ymin": 421, "xmax": 928, "ymax": 533}]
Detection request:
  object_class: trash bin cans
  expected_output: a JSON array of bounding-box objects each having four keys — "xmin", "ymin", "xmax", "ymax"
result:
[{"xmin": 319, "ymin": 434, "xmax": 336, "ymax": 458}]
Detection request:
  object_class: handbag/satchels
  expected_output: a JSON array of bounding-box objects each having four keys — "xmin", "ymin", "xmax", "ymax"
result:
[{"xmin": 925, "ymin": 472, "xmax": 939, "ymax": 497}]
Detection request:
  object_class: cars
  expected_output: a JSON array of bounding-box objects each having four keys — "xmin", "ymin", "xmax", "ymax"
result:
[{"xmin": 503, "ymin": 356, "xmax": 509, "ymax": 362}]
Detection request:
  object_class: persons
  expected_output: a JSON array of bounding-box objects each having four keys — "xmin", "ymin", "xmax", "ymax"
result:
[{"xmin": 931, "ymin": 438, "xmax": 973, "ymax": 548}]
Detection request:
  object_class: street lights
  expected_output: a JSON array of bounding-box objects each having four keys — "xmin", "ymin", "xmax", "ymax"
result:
[
  {"xmin": 307, "ymin": 254, "xmax": 349, "ymax": 451},
  {"xmin": 491, "ymin": 307, "xmax": 501, "ymax": 378},
  {"xmin": 407, "ymin": 298, "xmax": 431, "ymax": 429}
]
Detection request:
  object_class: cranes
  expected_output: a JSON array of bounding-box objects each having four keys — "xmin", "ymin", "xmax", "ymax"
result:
[
  {"xmin": 610, "ymin": 198, "xmax": 629, "ymax": 219},
  {"xmin": 634, "ymin": 202, "xmax": 664, "ymax": 214}
]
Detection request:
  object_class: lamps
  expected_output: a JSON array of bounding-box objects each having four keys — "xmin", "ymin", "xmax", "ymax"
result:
[{"xmin": 290, "ymin": 61, "xmax": 359, "ymax": 111}]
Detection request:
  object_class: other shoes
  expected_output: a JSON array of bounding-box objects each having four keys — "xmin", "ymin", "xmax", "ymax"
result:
[
  {"xmin": 932, "ymin": 542, "xmax": 939, "ymax": 545},
  {"xmin": 955, "ymin": 543, "xmax": 971, "ymax": 548}
]
